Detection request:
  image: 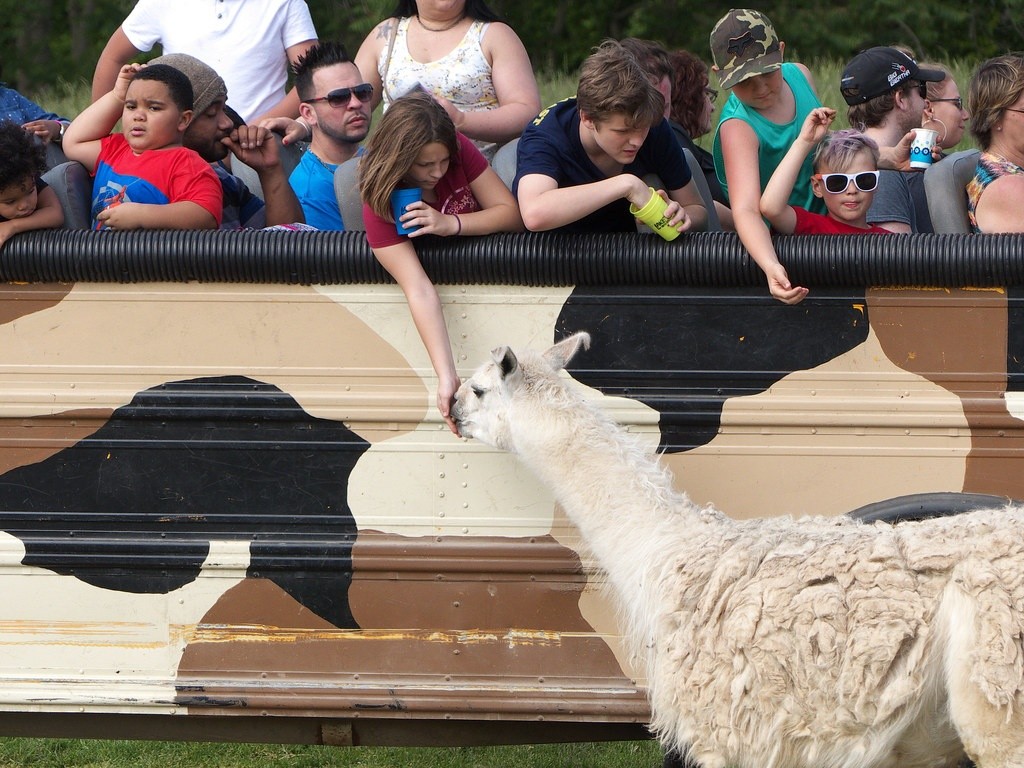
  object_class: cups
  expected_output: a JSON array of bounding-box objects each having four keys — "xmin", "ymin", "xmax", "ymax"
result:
[
  {"xmin": 909, "ymin": 128, "xmax": 939, "ymax": 169},
  {"xmin": 390, "ymin": 187, "xmax": 422, "ymax": 235},
  {"xmin": 629, "ymin": 187, "xmax": 683, "ymax": 242}
]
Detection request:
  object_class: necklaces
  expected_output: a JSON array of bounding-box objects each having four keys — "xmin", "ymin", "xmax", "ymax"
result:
[{"xmin": 415, "ymin": 14, "xmax": 467, "ymax": 32}]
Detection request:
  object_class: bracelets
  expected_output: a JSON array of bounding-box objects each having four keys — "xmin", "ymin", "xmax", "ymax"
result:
[
  {"xmin": 52, "ymin": 120, "xmax": 64, "ymax": 144},
  {"xmin": 295, "ymin": 116, "xmax": 312, "ymax": 141},
  {"xmin": 451, "ymin": 214, "xmax": 462, "ymax": 235}
]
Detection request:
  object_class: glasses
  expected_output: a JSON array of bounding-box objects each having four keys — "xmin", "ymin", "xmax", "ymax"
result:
[
  {"xmin": 906, "ymin": 82, "xmax": 928, "ymax": 99},
  {"xmin": 815, "ymin": 171, "xmax": 881, "ymax": 194},
  {"xmin": 702, "ymin": 87, "xmax": 720, "ymax": 103},
  {"xmin": 930, "ymin": 98, "xmax": 963, "ymax": 110},
  {"xmin": 304, "ymin": 82, "xmax": 374, "ymax": 108}
]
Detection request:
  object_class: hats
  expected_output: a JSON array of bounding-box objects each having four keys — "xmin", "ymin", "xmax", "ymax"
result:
[
  {"xmin": 146, "ymin": 53, "xmax": 226, "ymax": 119},
  {"xmin": 710, "ymin": 7, "xmax": 785, "ymax": 91},
  {"xmin": 840, "ymin": 46, "xmax": 945, "ymax": 105}
]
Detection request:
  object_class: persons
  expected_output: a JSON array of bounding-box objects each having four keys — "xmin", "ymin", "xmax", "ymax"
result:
[{"xmin": 0, "ymin": 0, "xmax": 1024, "ymax": 446}]
